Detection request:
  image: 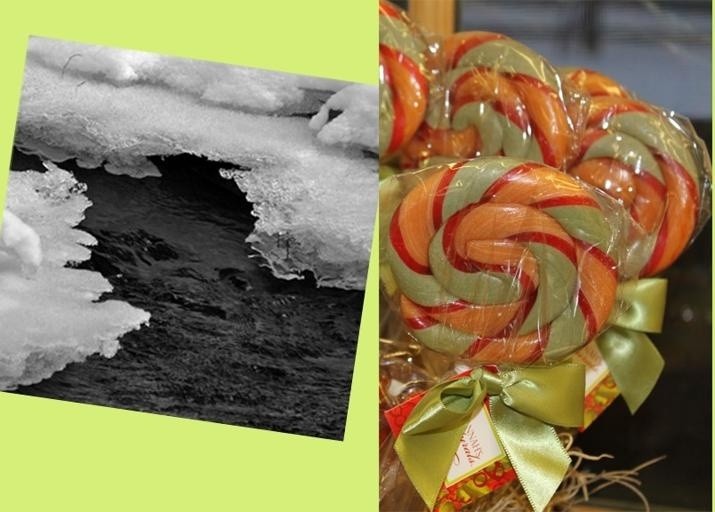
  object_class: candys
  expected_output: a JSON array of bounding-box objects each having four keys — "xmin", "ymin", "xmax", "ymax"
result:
[{"xmin": 378, "ymin": 0, "xmax": 701, "ymax": 367}]
